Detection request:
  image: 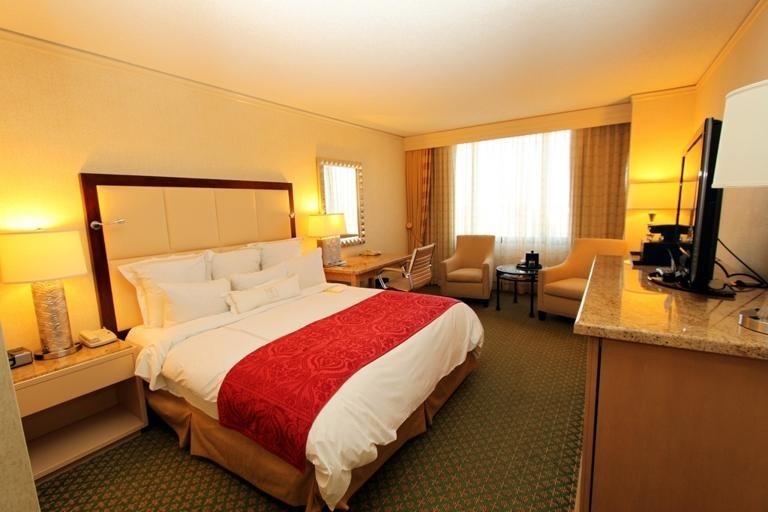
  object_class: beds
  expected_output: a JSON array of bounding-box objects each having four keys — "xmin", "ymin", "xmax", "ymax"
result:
[{"xmin": 79, "ymin": 173, "xmax": 485, "ymax": 511}]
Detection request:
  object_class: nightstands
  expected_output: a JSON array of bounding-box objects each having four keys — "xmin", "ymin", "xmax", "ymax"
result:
[{"xmin": 11, "ymin": 335, "xmax": 147, "ymax": 488}]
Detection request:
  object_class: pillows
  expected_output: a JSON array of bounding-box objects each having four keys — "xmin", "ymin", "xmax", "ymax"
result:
[
  {"xmin": 118, "ymin": 249, "xmax": 211, "ymax": 327},
  {"xmin": 229, "ymin": 262, "xmax": 289, "ymax": 289},
  {"xmin": 157, "ymin": 278, "xmax": 230, "ymax": 327},
  {"xmin": 207, "ymin": 246, "xmax": 261, "ymax": 278},
  {"xmin": 257, "ymin": 239, "xmax": 301, "ymax": 273},
  {"xmin": 223, "ymin": 275, "xmax": 299, "ymax": 315},
  {"xmin": 287, "ymin": 248, "xmax": 326, "ymax": 290}
]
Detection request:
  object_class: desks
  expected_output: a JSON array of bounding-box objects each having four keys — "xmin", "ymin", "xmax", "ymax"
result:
[
  {"xmin": 324, "ymin": 251, "xmax": 413, "ymax": 288},
  {"xmin": 574, "ymin": 253, "xmax": 768, "ymax": 512}
]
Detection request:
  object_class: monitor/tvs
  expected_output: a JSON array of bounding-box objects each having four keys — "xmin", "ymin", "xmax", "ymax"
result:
[{"xmin": 646, "ymin": 116, "xmax": 738, "ymax": 298}]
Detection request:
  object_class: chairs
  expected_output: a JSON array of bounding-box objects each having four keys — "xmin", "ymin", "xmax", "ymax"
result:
[
  {"xmin": 537, "ymin": 239, "xmax": 627, "ymax": 322},
  {"xmin": 439, "ymin": 235, "xmax": 496, "ymax": 307},
  {"xmin": 377, "ymin": 244, "xmax": 435, "ymax": 292}
]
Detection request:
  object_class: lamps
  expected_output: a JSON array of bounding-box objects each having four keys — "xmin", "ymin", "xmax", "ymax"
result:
[
  {"xmin": 305, "ymin": 213, "xmax": 345, "ymax": 267},
  {"xmin": 0, "ymin": 227, "xmax": 88, "ymax": 359},
  {"xmin": 711, "ymin": 81, "xmax": 764, "ymax": 335}
]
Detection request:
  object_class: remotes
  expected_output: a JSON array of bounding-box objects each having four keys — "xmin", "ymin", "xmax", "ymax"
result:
[{"xmin": 656, "ymin": 265, "xmax": 673, "ymax": 276}]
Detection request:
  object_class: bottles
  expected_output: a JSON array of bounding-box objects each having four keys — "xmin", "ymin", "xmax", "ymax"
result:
[{"xmin": 641, "ymin": 213, "xmax": 660, "ymax": 252}]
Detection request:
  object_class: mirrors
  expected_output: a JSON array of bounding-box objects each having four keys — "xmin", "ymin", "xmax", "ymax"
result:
[{"xmin": 317, "ymin": 157, "xmax": 365, "ymax": 246}]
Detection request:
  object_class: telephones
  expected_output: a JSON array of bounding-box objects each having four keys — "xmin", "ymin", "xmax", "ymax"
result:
[{"xmin": 78, "ymin": 328, "xmax": 118, "ymax": 348}]
